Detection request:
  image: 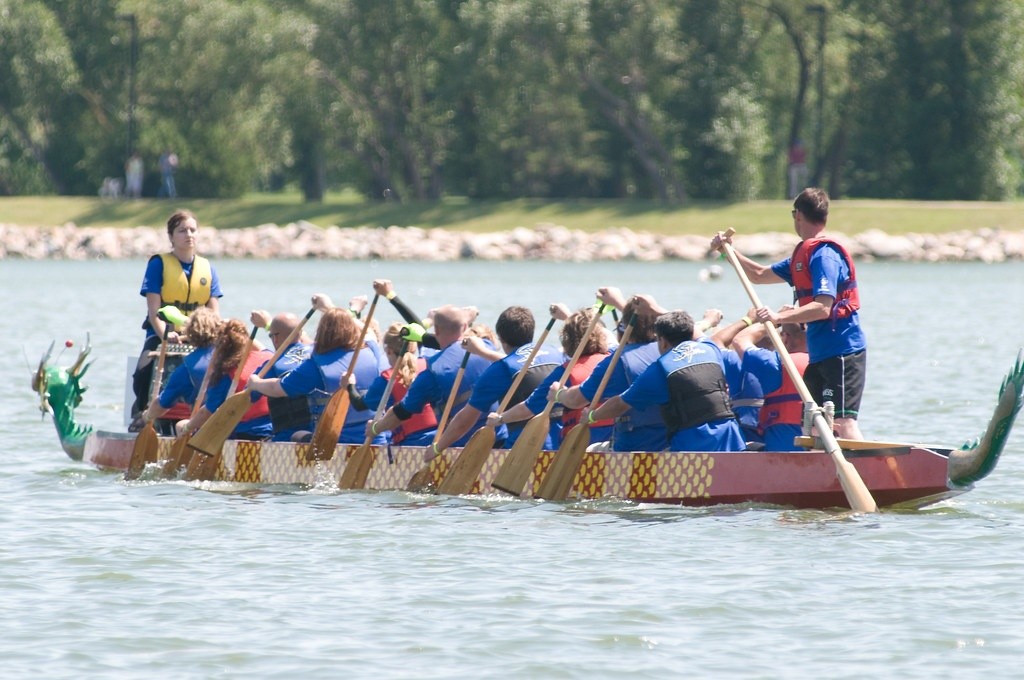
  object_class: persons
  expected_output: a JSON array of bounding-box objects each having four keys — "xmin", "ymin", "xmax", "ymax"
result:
[
  {"xmin": 711, "ymin": 187, "xmax": 866, "ymax": 441},
  {"xmin": 158, "ymin": 149, "xmax": 177, "ymax": 196},
  {"xmin": 125, "ymin": 149, "xmax": 143, "ymax": 197},
  {"xmin": 788, "ymin": 139, "xmax": 808, "ymax": 198},
  {"xmin": 128, "ymin": 212, "xmax": 223, "ymax": 434},
  {"xmin": 142, "ymin": 279, "xmax": 810, "ymax": 450}
]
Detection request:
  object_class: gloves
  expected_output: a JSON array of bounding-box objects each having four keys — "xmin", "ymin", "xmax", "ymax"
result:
[
  {"xmin": 399, "ymin": 323, "xmax": 427, "ymax": 341},
  {"xmin": 157, "ymin": 305, "xmax": 187, "ymax": 326}
]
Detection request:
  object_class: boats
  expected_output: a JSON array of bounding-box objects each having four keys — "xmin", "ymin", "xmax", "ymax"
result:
[{"xmin": 29, "ymin": 332, "xmax": 1024, "ymax": 514}]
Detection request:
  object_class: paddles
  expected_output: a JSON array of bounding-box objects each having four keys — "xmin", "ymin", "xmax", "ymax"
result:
[
  {"xmin": 710, "ymin": 227, "xmax": 882, "ymax": 514},
  {"xmin": 183, "ymin": 326, "xmax": 258, "ymax": 482},
  {"xmin": 491, "ymin": 302, "xmax": 607, "ymax": 496},
  {"xmin": 534, "ymin": 297, "xmax": 640, "ymax": 500},
  {"xmin": 406, "ymin": 339, "xmax": 471, "ymax": 491},
  {"xmin": 305, "ymin": 284, "xmax": 379, "ymax": 461},
  {"xmin": 337, "ymin": 328, "xmax": 410, "ymax": 490},
  {"xmin": 186, "ymin": 296, "xmax": 317, "ymax": 457},
  {"xmin": 123, "ymin": 323, "xmax": 170, "ymax": 481},
  {"xmin": 434, "ymin": 306, "xmax": 556, "ymax": 496},
  {"xmin": 159, "ymin": 348, "xmax": 216, "ymax": 479}
]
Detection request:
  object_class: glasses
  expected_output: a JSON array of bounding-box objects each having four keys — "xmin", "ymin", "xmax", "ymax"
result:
[{"xmin": 792, "ymin": 209, "xmax": 800, "ymax": 219}]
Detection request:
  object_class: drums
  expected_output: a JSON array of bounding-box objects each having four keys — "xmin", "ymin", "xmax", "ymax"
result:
[{"xmin": 146, "ymin": 343, "xmax": 197, "ymax": 436}]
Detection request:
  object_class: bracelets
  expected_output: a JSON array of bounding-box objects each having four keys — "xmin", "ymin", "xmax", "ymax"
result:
[
  {"xmin": 433, "ymin": 442, "xmax": 441, "ymax": 454},
  {"xmin": 146, "ymin": 416, "xmax": 151, "ymax": 421},
  {"xmin": 386, "ymin": 291, "xmax": 396, "ymax": 300},
  {"xmin": 372, "ymin": 422, "xmax": 378, "ymax": 434},
  {"xmin": 588, "ymin": 410, "xmax": 597, "ymax": 422},
  {"xmin": 556, "ymin": 389, "xmax": 562, "ymax": 401},
  {"xmin": 185, "ymin": 424, "xmax": 191, "ymax": 432},
  {"xmin": 742, "ymin": 316, "xmax": 752, "ymax": 326},
  {"xmin": 265, "ymin": 323, "xmax": 270, "ymax": 331},
  {"xmin": 720, "ymin": 253, "xmax": 726, "ymax": 258}
]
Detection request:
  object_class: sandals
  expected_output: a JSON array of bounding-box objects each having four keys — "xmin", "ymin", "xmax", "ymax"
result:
[{"xmin": 129, "ymin": 419, "xmax": 143, "ymax": 433}]
